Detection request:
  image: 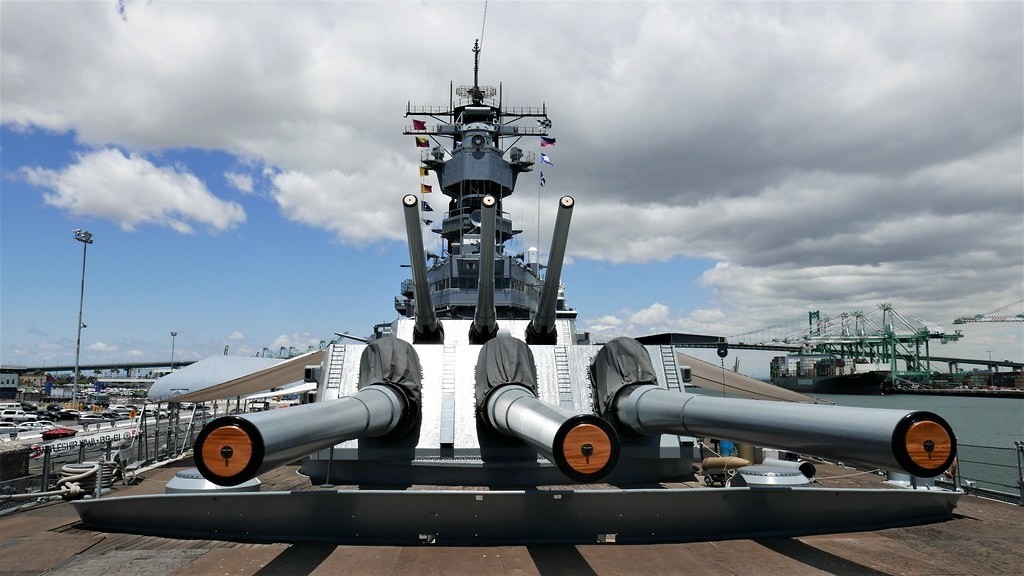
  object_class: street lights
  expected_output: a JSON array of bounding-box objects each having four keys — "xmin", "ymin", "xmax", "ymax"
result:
[
  {"xmin": 72, "ymin": 228, "xmax": 94, "ymax": 409},
  {"xmin": 170, "ymin": 331, "xmax": 178, "ymax": 372}
]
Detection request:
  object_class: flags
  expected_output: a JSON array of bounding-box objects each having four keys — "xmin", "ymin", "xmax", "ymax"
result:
[
  {"xmin": 537, "ymin": 117, "xmax": 552, "ymax": 129},
  {"xmin": 419, "ymin": 166, "xmax": 430, "ymax": 176},
  {"xmin": 541, "ymin": 136, "xmax": 556, "ymax": 147},
  {"xmin": 415, "ymin": 136, "xmax": 429, "ymax": 147},
  {"xmin": 413, "ymin": 119, "xmax": 426, "ymax": 130},
  {"xmin": 421, "ymin": 183, "xmax": 432, "ymax": 193},
  {"xmin": 423, "ymin": 218, "xmax": 433, "ymax": 226},
  {"xmin": 540, "ymin": 171, "xmax": 546, "ymax": 187},
  {"xmin": 541, "ymin": 153, "xmax": 554, "ymax": 167},
  {"xmin": 421, "ymin": 200, "xmax": 433, "ymax": 211}
]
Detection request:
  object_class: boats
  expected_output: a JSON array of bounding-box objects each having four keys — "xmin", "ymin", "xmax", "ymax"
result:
[{"xmin": 29, "ymin": 428, "xmax": 143, "ymax": 463}]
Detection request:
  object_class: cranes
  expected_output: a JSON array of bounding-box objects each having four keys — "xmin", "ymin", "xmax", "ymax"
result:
[
  {"xmin": 763, "ymin": 300, "xmax": 965, "ymax": 387},
  {"xmin": 955, "ymin": 299, "xmax": 1024, "ymax": 324}
]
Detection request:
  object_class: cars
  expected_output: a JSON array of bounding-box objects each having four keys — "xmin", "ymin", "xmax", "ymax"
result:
[
  {"xmin": 230, "ymin": 408, "xmax": 244, "ymax": 415},
  {"xmin": 0, "ymin": 397, "xmax": 211, "ymax": 436}
]
